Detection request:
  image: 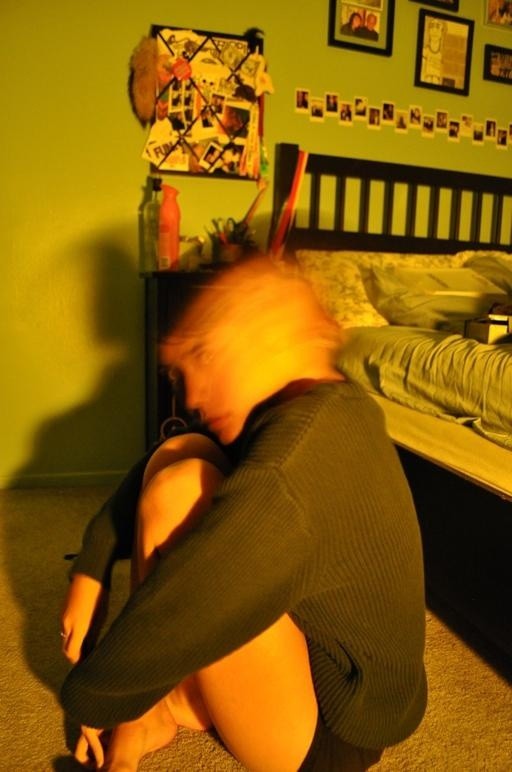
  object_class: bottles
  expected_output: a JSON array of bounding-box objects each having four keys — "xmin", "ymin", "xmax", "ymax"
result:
[{"xmin": 142, "ymin": 177, "xmax": 161, "ymax": 267}]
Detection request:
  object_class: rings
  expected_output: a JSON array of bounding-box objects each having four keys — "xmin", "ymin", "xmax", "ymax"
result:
[{"xmin": 61, "ymin": 634, "xmax": 67, "ymax": 639}]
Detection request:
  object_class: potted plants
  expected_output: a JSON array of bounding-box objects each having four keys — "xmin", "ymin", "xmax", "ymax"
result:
[{"xmin": 206, "ymin": 213, "xmax": 258, "ymax": 268}]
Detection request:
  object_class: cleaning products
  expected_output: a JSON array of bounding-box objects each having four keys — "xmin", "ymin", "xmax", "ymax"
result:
[
  {"xmin": 158, "ymin": 183, "xmax": 183, "ymax": 272},
  {"xmin": 141, "ymin": 176, "xmax": 163, "ymax": 270}
]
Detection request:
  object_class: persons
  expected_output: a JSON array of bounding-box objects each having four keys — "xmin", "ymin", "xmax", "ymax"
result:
[
  {"xmin": 420, "ymin": 19, "xmax": 448, "ymax": 84},
  {"xmin": 355, "ymin": 14, "xmax": 378, "ymax": 40},
  {"xmin": 60, "ymin": 252, "xmax": 429, "ymax": 772},
  {"xmin": 341, "ymin": 12, "xmax": 362, "ymax": 35}
]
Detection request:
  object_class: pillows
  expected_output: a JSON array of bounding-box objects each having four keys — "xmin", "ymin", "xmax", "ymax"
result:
[{"xmin": 294, "ymin": 245, "xmax": 512, "ymax": 331}]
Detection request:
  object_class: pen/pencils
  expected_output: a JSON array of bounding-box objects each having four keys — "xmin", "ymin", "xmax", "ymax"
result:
[{"xmin": 211, "ymin": 216, "xmax": 252, "ymax": 244}]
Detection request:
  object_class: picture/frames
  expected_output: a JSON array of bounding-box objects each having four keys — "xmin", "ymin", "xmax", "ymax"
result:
[{"xmin": 325, "ymin": 2, "xmax": 512, "ymax": 97}]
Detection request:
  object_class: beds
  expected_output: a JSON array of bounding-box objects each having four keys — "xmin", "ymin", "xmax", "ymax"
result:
[{"xmin": 266, "ymin": 140, "xmax": 512, "ymax": 690}]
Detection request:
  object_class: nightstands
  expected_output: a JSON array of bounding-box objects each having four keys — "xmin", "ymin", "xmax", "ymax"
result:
[{"xmin": 142, "ymin": 264, "xmax": 256, "ymax": 470}]
